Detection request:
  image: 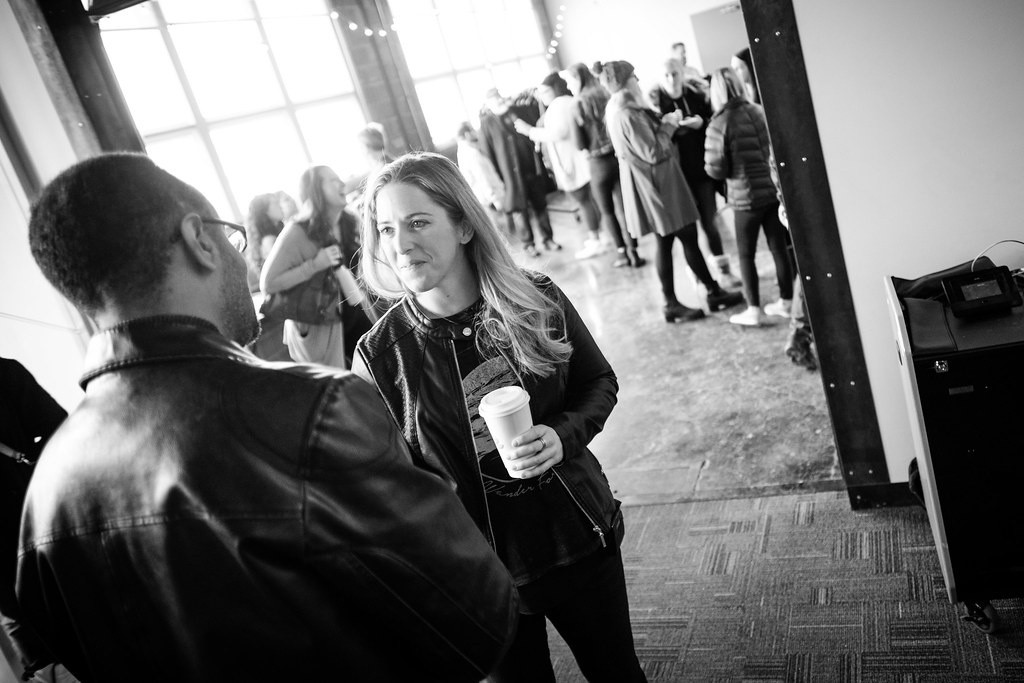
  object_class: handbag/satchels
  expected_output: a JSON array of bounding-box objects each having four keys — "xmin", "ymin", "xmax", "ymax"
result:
[{"xmin": 259, "ymin": 266, "xmax": 340, "ymax": 326}]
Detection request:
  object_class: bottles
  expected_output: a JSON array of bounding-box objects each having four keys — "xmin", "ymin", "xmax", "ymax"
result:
[{"xmin": 332, "ymin": 260, "xmax": 362, "ymax": 306}]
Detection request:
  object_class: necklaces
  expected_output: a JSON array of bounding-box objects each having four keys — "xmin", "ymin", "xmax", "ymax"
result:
[{"xmin": 431, "ymin": 312, "xmax": 472, "ymax": 337}]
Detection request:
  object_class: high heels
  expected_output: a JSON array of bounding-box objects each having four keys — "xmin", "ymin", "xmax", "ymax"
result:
[
  {"xmin": 707, "ymin": 281, "xmax": 746, "ymax": 312},
  {"xmin": 664, "ymin": 288, "xmax": 704, "ymax": 324}
]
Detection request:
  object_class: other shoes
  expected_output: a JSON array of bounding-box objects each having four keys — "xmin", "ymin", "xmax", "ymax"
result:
[
  {"xmin": 729, "ymin": 306, "xmax": 760, "ymax": 326},
  {"xmin": 624, "ymin": 247, "xmax": 646, "ymax": 268},
  {"xmin": 763, "ymin": 298, "xmax": 792, "ymax": 318},
  {"xmin": 527, "ymin": 245, "xmax": 541, "ymax": 257},
  {"xmin": 545, "ymin": 239, "xmax": 561, "ymax": 251},
  {"xmin": 575, "ymin": 239, "xmax": 613, "ymax": 260},
  {"xmin": 716, "ymin": 256, "xmax": 743, "ymax": 288}
]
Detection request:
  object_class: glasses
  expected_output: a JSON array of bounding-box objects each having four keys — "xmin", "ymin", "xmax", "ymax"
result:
[{"xmin": 169, "ymin": 218, "xmax": 248, "ymax": 254}]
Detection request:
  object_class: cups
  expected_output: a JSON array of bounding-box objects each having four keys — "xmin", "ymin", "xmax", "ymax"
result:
[{"xmin": 478, "ymin": 385, "xmax": 543, "ymax": 480}]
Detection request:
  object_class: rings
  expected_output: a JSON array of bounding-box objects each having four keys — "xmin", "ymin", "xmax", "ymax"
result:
[{"xmin": 539, "ymin": 438, "xmax": 546, "ymax": 447}]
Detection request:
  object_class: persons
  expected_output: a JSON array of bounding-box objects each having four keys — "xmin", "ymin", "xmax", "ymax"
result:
[
  {"xmin": 260, "ymin": 167, "xmax": 392, "ymax": 369},
  {"xmin": 730, "ymin": 50, "xmax": 761, "ymax": 103},
  {"xmin": 358, "ymin": 126, "xmax": 393, "ymax": 165},
  {"xmin": 515, "ymin": 72, "xmax": 614, "ymax": 261},
  {"xmin": 768, "ymin": 145, "xmax": 815, "ymax": 370},
  {"xmin": 566, "ymin": 63, "xmax": 648, "ymax": 268},
  {"xmin": 0, "ymin": 152, "xmax": 517, "ymax": 683},
  {"xmin": 650, "ymin": 60, "xmax": 742, "ymax": 289},
  {"xmin": 478, "ymin": 88, "xmax": 561, "ymax": 257},
  {"xmin": 248, "ymin": 190, "xmax": 298, "ymax": 258},
  {"xmin": 704, "ymin": 67, "xmax": 792, "ymax": 325},
  {"xmin": 672, "ymin": 42, "xmax": 702, "ymax": 76},
  {"xmin": 594, "ymin": 60, "xmax": 745, "ymax": 323},
  {"xmin": 351, "ymin": 153, "xmax": 648, "ymax": 683},
  {"xmin": 0, "ymin": 356, "xmax": 68, "ymax": 608},
  {"xmin": 455, "ymin": 124, "xmax": 507, "ymax": 220}
]
